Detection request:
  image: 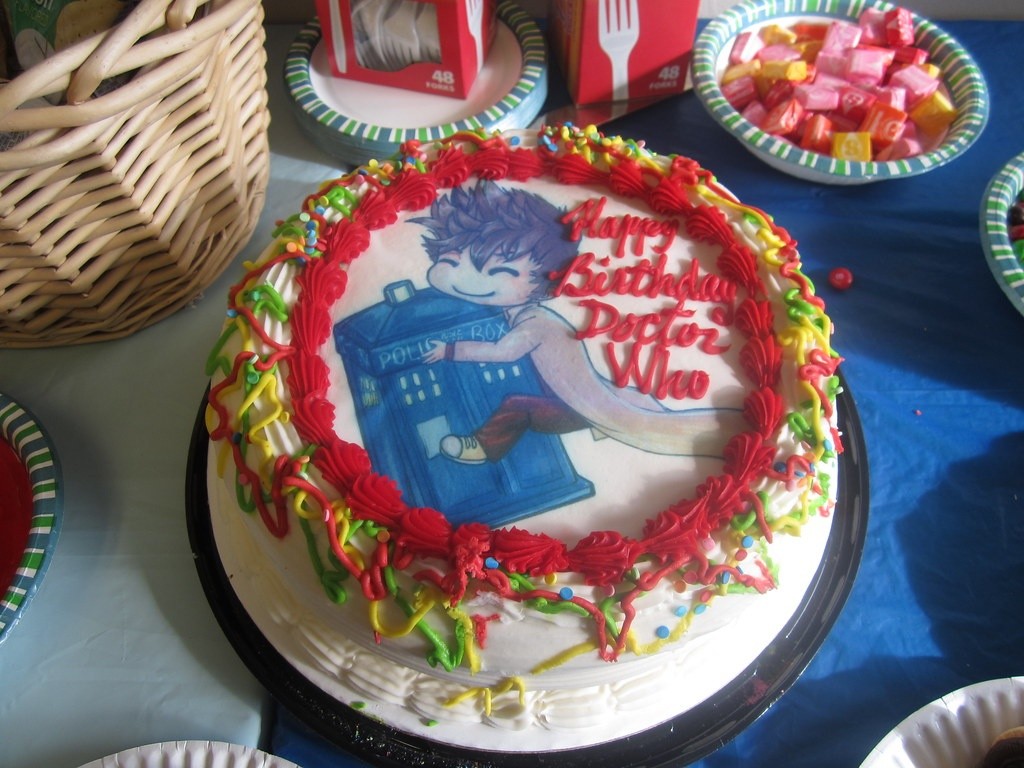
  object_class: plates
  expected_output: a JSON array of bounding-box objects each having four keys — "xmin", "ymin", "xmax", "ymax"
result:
[
  {"xmin": 858, "ymin": 676, "xmax": 1024, "ymax": 768},
  {"xmin": 78, "ymin": 740, "xmax": 302, "ymax": 768},
  {"xmin": 0, "ymin": 393, "xmax": 62, "ymax": 641},
  {"xmin": 283, "ymin": 0, "xmax": 549, "ymax": 170},
  {"xmin": 691, "ymin": 0, "xmax": 990, "ymax": 185}
]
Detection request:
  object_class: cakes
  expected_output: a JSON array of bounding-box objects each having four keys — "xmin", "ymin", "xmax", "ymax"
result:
[{"xmin": 198, "ymin": 120, "xmax": 843, "ymax": 725}]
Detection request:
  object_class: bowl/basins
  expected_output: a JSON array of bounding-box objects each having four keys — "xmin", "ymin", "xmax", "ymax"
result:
[{"xmin": 980, "ymin": 153, "xmax": 1024, "ymax": 316}]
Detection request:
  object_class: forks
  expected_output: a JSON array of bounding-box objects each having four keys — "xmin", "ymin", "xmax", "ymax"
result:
[
  {"xmin": 597, "ymin": 0, "xmax": 641, "ymax": 100},
  {"xmin": 349, "ymin": 0, "xmax": 446, "ymax": 71}
]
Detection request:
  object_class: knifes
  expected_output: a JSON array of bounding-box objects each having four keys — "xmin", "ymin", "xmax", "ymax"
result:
[{"xmin": 465, "ymin": 0, "xmax": 485, "ymax": 73}]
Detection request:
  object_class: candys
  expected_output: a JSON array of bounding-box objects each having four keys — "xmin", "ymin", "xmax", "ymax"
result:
[{"xmin": 719, "ymin": 7, "xmax": 961, "ymax": 162}]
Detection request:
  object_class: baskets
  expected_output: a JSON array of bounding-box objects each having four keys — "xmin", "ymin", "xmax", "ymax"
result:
[{"xmin": 0, "ymin": 0, "xmax": 268, "ymax": 349}]
